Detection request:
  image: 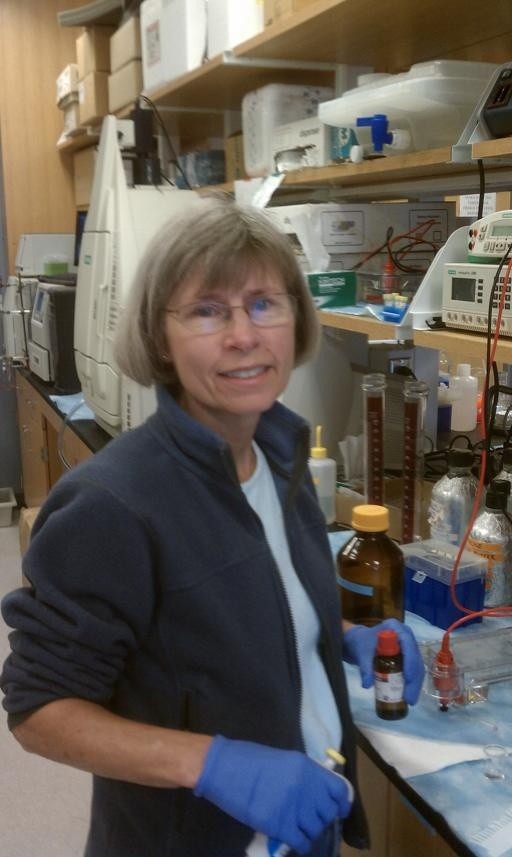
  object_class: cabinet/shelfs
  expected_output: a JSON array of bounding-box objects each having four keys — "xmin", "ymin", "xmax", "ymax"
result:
[{"xmin": 14, "ymin": 0, "xmax": 512, "ymax": 857}]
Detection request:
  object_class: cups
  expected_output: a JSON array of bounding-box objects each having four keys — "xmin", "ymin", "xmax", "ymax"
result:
[{"xmin": 482, "ymin": 743, "xmax": 508, "ymax": 783}]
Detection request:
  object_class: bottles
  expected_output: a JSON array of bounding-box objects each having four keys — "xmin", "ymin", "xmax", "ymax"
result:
[
  {"xmin": 370, "ymin": 628, "xmax": 411, "ymax": 718},
  {"xmin": 305, "ymin": 424, "xmax": 336, "ymax": 528},
  {"xmin": 335, "ymin": 502, "xmax": 409, "ymax": 630},
  {"xmin": 437, "ymin": 382, "xmax": 451, "ymax": 431},
  {"xmin": 428, "ymin": 449, "xmax": 512, "ymax": 609},
  {"xmin": 449, "ymin": 361, "xmax": 478, "ymax": 431}
]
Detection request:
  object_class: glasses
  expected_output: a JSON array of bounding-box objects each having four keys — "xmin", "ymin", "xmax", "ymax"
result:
[{"xmin": 162, "ymin": 293, "xmax": 297, "ymax": 333}]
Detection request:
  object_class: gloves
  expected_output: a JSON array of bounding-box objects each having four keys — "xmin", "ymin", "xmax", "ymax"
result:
[
  {"xmin": 345, "ymin": 618, "xmax": 423, "ymax": 705},
  {"xmin": 193, "ymin": 735, "xmax": 356, "ymax": 856}
]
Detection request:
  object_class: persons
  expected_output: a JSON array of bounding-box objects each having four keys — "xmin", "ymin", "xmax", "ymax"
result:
[{"xmin": 0, "ymin": 186, "xmax": 427, "ymax": 857}]
